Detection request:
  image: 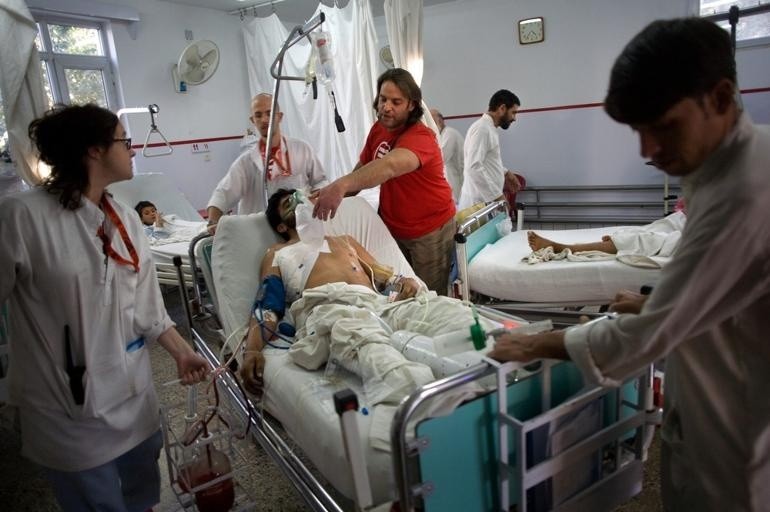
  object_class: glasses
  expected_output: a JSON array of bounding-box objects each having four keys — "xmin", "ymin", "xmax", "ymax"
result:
[{"xmin": 108, "ymin": 138, "xmax": 132, "ymax": 151}]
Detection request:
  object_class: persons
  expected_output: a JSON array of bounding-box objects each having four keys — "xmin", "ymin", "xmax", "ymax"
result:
[
  {"xmin": 240, "ymin": 188, "xmax": 494, "ymax": 403},
  {"xmin": 308, "ymin": 68, "xmax": 456, "ymax": 296},
  {"xmin": 458, "ymin": 89, "xmax": 521, "ymax": 212},
  {"xmin": 528, "ymin": 211, "xmax": 686, "ymax": 253},
  {"xmin": 206, "ymin": 92, "xmax": 330, "ymax": 234},
  {"xmin": 430, "ymin": 108, "xmax": 464, "ymax": 200},
  {"xmin": 135, "ymin": 200, "xmax": 167, "ymax": 239},
  {"xmin": 486, "ymin": 15, "xmax": 770, "ymax": 512},
  {"xmin": 0, "ymin": 102, "xmax": 212, "ymax": 512}
]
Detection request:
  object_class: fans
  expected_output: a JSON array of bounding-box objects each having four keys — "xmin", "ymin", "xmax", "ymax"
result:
[{"xmin": 173, "ymin": 39, "xmax": 221, "ymax": 94}]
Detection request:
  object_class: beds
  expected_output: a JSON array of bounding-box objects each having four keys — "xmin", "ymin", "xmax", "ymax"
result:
[
  {"xmin": 455, "ymin": 195, "xmax": 686, "ymax": 325},
  {"xmin": 105, "ymin": 173, "xmax": 209, "ymax": 289},
  {"xmin": 171, "ymin": 194, "xmax": 663, "ymax": 510}
]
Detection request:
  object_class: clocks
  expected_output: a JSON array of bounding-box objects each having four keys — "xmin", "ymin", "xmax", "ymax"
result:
[{"xmin": 518, "ymin": 16, "xmax": 544, "ymax": 44}]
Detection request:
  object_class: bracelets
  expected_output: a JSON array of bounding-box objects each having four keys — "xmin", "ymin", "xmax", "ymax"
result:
[
  {"xmin": 505, "ymin": 169, "xmax": 510, "ymax": 176},
  {"xmin": 207, "ymin": 219, "xmax": 218, "ymax": 227}
]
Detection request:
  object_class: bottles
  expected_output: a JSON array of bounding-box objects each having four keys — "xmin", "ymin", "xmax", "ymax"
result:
[{"xmin": 176, "ymin": 414, "xmax": 235, "ymax": 511}]
